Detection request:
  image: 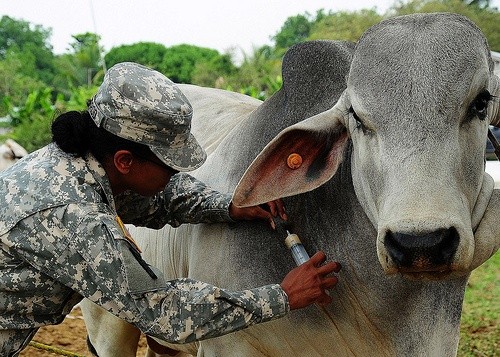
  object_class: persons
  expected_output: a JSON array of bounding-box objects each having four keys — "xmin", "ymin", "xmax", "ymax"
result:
[{"xmin": 0, "ymin": 61, "xmax": 342, "ymax": 357}]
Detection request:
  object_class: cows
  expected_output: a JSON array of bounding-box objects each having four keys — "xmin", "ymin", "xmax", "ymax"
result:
[{"xmin": 80, "ymin": 10, "xmax": 500, "ymax": 357}]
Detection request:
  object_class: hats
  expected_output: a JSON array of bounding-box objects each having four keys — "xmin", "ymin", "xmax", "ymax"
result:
[{"xmin": 86, "ymin": 61, "xmax": 208, "ymax": 173}]
